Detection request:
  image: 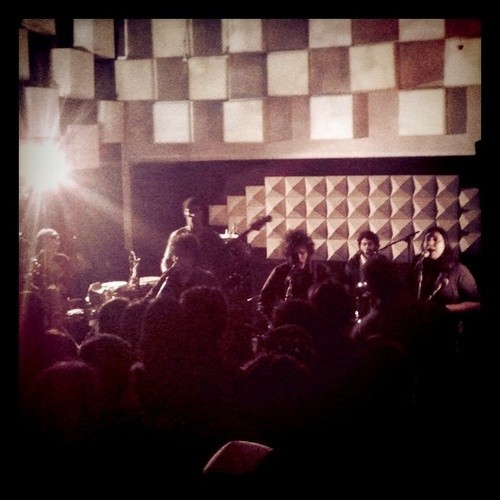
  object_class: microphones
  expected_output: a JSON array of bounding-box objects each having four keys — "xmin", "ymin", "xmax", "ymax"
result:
[
  {"xmin": 250, "ymin": 214, "xmax": 271, "ymax": 231},
  {"xmin": 285, "ymin": 263, "xmax": 298, "ymax": 282},
  {"xmin": 427, "ymin": 277, "xmax": 450, "ymax": 300},
  {"xmin": 411, "ymin": 251, "xmax": 431, "ymax": 270}
]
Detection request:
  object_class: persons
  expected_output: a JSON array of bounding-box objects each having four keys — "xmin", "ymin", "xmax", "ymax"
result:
[{"xmin": 22, "ymin": 196, "xmax": 482, "ymax": 433}]
